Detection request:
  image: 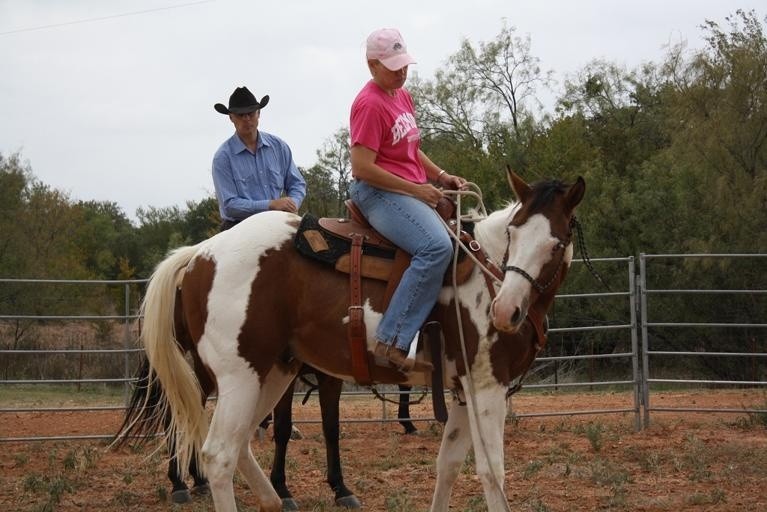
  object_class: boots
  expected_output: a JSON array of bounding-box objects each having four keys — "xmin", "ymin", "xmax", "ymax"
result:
[{"xmin": 374, "ymin": 341, "xmax": 434, "ymax": 373}]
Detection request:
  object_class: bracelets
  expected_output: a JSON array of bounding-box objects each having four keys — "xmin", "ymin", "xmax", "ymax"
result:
[{"xmin": 434, "ymin": 169, "xmax": 445, "ymax": 183}]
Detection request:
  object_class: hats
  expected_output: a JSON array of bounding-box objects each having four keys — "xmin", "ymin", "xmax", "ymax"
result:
[
  {"xmin": 366, "ymin": 29, "xmax": 417, "ymax": 72},
  {"xmin": 214, "ymin": 86, "xmax": 269, "ymax": 114}
]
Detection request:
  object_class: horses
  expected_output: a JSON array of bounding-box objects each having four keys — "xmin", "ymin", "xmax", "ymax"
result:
[
  {"xmin": 139, "ymin": 161, "xmax": 587, "ymax": 512},
  {"xmin": 106, "ymin": 160, "xmax": 586, "ymax": 512}
]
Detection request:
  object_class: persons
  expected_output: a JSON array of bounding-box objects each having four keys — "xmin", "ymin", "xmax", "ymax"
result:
[
  {"xmin": 347, "ymin": 25, "xmax": 473, "ymax": 373},
  {"xmin": 211, "ymin": 84, "xmax": 307, "ymax": 232}
]
